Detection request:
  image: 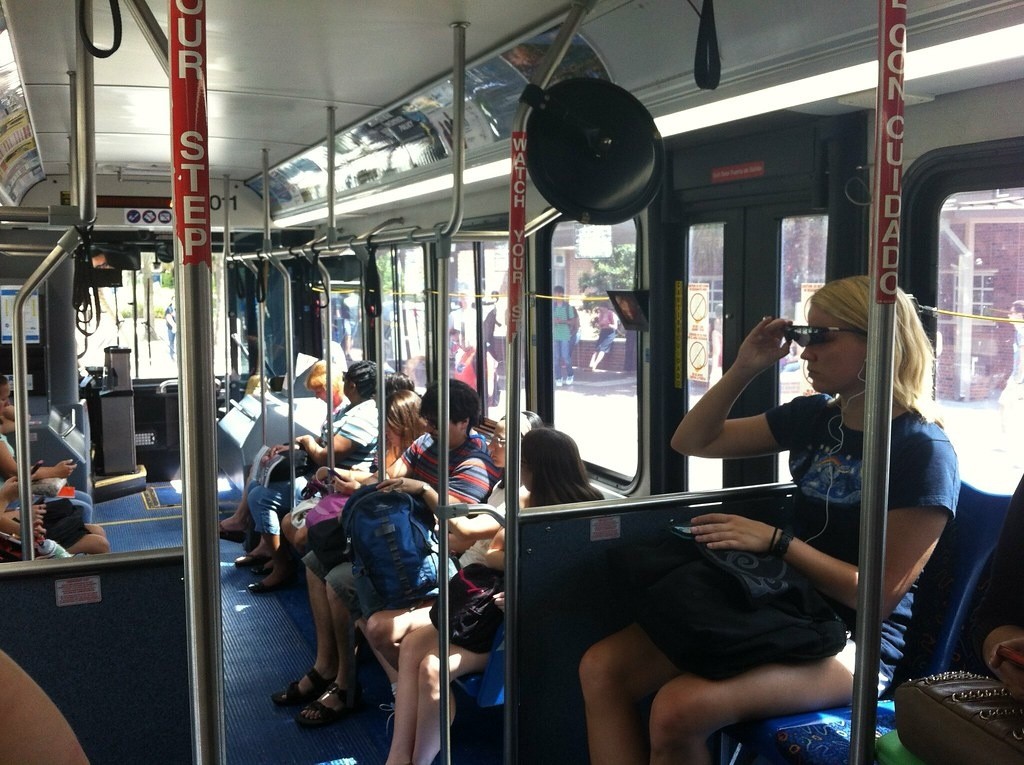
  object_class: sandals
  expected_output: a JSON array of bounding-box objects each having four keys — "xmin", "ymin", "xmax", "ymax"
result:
[
  {"xmin": 272, "ymin": 666, "xmax": 340, "ymax": 704},
  {"xmin": 293, "ymin": 683, "xmax": 367, "ymax": 728}
]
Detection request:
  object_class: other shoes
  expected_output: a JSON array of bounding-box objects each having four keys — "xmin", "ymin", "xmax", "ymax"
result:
[
  {"xmin": 566, "ymin": 375, "xmax": 574, "ymax": 386},
  {"xmin": 555, "ymin": 376, "xmax": 563, "ymax": 387},
  {"xmin": 250, "ymin": 564, "xmax": 274, "ymax": 573},
  {"xmin": 249, "ymin": 575, "xmax": 287, "ymax": 593}
]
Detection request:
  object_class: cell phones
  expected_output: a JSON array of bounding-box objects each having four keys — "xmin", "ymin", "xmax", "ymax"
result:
[
  {"xmin": 672, "ymin": 526, "xmax": 697, "ymax": 539},
  {"xmin": 997, "ymin": 645, "xmax": 1024, "ymax": 669},
  {"xmin": 31, "ymin": 460, "xmax": 44, "ymax": 475},
  {"xmin": 327, "ymin": 467, "xmax": 342, "ymax": 482}
]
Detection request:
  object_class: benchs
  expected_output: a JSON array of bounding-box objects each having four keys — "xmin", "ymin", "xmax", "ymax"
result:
[{"xmin": 722, "ymin": 479, "xmax": 1014, "ymax": 765}]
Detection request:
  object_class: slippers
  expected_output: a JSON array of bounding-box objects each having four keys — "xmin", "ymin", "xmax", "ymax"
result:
[
  {"xmin": 219, "ymin": 520, "xmax": 247, "ymax": 544},
  {"xmin": 234, "ymin": 554, "xmax": 272, "ymax": 567}
]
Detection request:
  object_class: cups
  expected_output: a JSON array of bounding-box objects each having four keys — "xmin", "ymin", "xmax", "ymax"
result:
[{"xmin": 307, "ymin": 466, "xmax": 331, "ymax": 493}]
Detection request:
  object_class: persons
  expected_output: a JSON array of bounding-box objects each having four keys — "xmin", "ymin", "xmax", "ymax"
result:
[
  {"xmin": 340, "ymin": 292, "xmax": 361, "ymax": 361},
  {"xmin": 363, "ymin": 410, "xmax": 604, "ymax": 765},
  {"xmin": 0, "ymin": 375, "xmax": 111, "ymax": 564},
  {"xmin": 552, "ymin": 286, "xmax": 575, "ymax": 387},
  {"xmin": 582, "ymin": 301, "xmax": 618, "ymax": 372},
  {"xmin": 162, "ymin": 295, "xmax": 177, "ymax": 361},
  {"xmin": 270, "ymin": 378, "xmax": 505, "ymax": 728},
  {"xmin": 220, "ymin": 355, "xmax": 432, "ymax": 594},
  {"xmin": 578, "ymin": 267, "xmax": 962, "ymax": 764},
  {"xmin": 995, "ymin": 298, "xmax": 1024, "ymax": 459},
  {"xmin": 450, "ymin": 289, "xmax": 502, "ymax": 408}
]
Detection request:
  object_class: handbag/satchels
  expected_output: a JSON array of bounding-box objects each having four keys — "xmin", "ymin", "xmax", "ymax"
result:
[
  {"xmin": 428, "ymin": 561, "xmax": 510, "ymax": 653},
  {"xmin": 625, "ymin": 521, "xmax": 847, "ymax": 682},
  {"xmin": 896, "ymin": 670, "xmax": 1024, "ymax": 765},
  {"xmin": 267, "ymin": 442, "xmax": 314, "ymax": 480}
]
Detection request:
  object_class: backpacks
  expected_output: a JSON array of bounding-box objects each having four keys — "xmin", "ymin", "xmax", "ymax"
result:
[
  {"xmin": 473, "ymin": 350, "xmax": 500, "ymax": 407},
  {"xmin": 339, "ymin": 482, "xmax": 441, "ymax": 611},
  {"xmin": 566, "ymin": 306, "xmax": 578, "ymax": 335}
]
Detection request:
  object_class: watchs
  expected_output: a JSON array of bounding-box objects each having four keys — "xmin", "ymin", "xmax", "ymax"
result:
[{"xmin": 420, "ymin": 482, "xmax": 429, "ymax": 498}]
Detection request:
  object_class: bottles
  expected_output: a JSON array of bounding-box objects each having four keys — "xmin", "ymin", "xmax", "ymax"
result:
[{"xmin": 38, "ymin": 539, "xmax": 71, "ymax": 557}]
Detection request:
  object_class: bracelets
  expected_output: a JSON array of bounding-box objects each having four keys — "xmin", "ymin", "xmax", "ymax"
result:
[
  {"xmin": 774, "ymin": 523, "xmax": 795, "ymax": 554},
  {"xmin": 769, "ymin": 527, "xmax": 778, "ymax": 552}
]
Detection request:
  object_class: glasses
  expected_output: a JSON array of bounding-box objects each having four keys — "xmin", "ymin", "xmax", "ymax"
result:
[
  {"xmin": 423, "ymin": 417, "xmax": 440, "ymax": 430},
  {"xmin": 783, "ymin": 324, "xmax": 858, "ymax": 346},
  {"xmin": 485, "ymin": 433, "xmax": 506, "ymax": 447}
]
